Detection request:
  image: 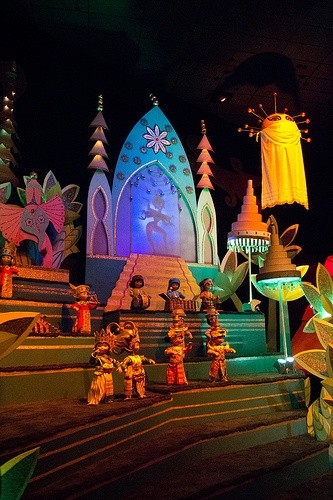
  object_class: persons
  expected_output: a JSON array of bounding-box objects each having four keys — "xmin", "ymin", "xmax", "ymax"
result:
[
  {"xmin": 67, "ymin": 276, "xmax": 239, "ymax": 404},
  {"xmin": 1, "ymin": 242, "xmax": 21, "ymax": 299}
]
